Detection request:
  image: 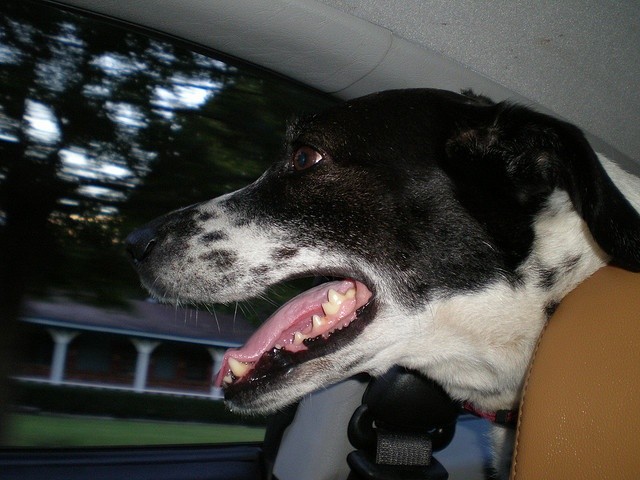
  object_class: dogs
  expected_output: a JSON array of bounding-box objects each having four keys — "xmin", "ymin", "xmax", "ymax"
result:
[{"xmin": 120, "ymin": 84, "xmax": 640, "ymax": 480}]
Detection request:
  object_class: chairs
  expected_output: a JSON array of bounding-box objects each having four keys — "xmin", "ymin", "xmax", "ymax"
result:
[
  {"xmin": 511, "ymin": 265, "xmax": 640, "ymax": 480},
  {"xmin": 262, "ymin": 364, "xmax": 512, "ymax": 479}
]
